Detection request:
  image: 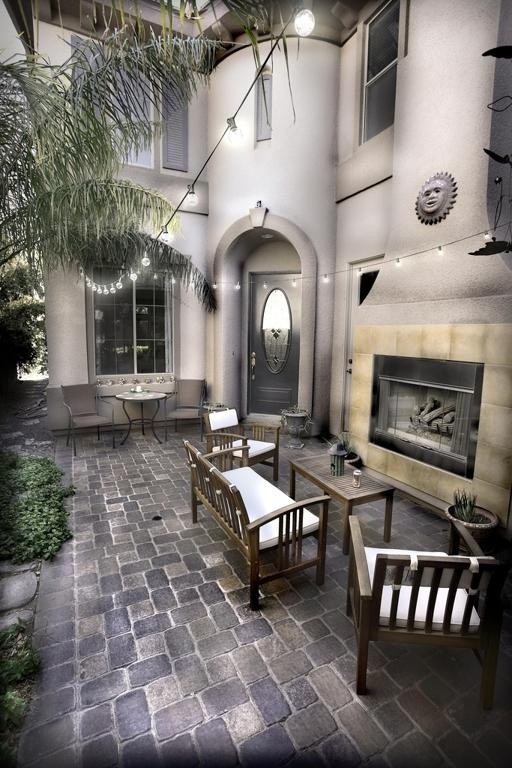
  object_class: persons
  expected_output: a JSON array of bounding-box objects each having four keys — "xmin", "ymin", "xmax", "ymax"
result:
[{"xmin": 418, "ymin": 179, "xmax": 448, "ymax": 213}]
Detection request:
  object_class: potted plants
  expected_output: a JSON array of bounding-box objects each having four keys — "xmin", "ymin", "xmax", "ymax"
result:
[{"xmin": 444, "ymin": 485, "xmax": 499, "ymax": 549}]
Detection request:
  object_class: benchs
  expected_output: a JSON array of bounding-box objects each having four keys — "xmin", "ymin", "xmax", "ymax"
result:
[{"xmin": 181, "ymin": 440, "xmax": 326, "ymax": 614}]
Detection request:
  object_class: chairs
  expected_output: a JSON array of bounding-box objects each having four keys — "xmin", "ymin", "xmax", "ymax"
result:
[
  {"xmin": 186, "ymin": 399, "xmax": 282, "ymax": 482},
  {"xmin": 61, "ymin": 376, "xmax": 211, "ymax": 459},
  {"xmin": 342, "ymin": 513, "xmax": 506, "ymax": 710}
]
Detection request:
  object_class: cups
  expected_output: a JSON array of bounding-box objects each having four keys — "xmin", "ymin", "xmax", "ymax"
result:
[{"xmin": 137, "ymin": 386, "xmax": 141, "ymax": 391}]
